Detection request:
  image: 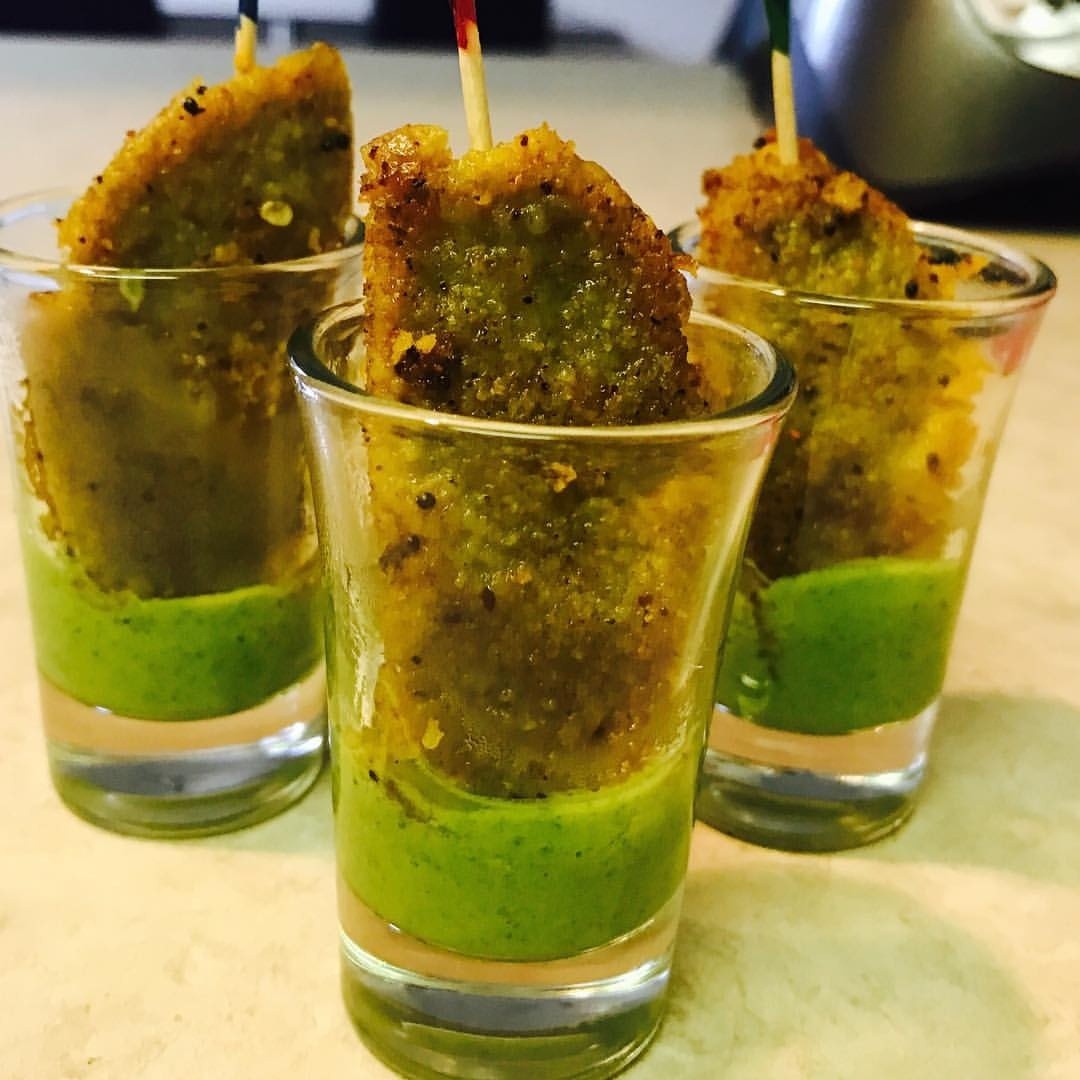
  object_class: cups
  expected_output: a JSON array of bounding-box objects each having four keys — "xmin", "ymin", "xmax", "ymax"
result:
[{"xmin": 3, "ymin": 199, "xmax": 1058, "ymax": 1080}]
